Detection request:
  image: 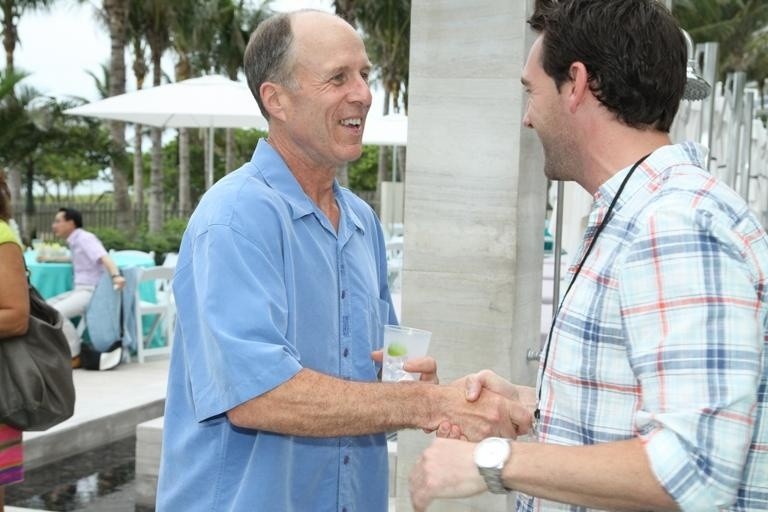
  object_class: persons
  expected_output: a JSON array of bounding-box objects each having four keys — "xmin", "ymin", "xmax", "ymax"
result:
[
  {"xmin": 0, "ymin": 154, "xmax": 32, "ymax": 512},
  {"xmin": 407, "ymin": 0, "xmax": 766, "ymax": 512},
  {"xmin": 35, "ymin": 208, "xmax": 127, "ymax": 368},
  {"xmin": 148, "ymin": 6, "xmax": 535, "ymax": 512}
]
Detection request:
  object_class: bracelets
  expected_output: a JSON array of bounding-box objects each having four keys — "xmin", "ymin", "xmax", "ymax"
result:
[{"xmin": 110, "ymin": 272, "xmax": 121, "ymax": 278}]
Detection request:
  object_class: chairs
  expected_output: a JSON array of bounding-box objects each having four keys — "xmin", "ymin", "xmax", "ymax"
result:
[
  {"xmin": 130, "ymin": 265, "xmax": 180, "ymax": 366},
  {"xmin": 158, "ymin": 250, "xmax": 180, "ymax": 351}
]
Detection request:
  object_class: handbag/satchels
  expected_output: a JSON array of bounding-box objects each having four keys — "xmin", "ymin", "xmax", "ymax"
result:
[
  {"xmin": 77, "ymin": 340, "xmax": 125, "ymax": 373},
  {"xmin": 0, "ymin": 258, "xmax": 77, "ymax": 433}
]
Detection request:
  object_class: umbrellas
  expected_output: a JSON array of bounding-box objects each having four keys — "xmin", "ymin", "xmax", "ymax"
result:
[
  {"xmin": 361, "ymin": 61, "xmax": 405, "ymax": 151},
  {"xmin": 58, "ymin": 71, "xmax": 269, "ymax": 199}
]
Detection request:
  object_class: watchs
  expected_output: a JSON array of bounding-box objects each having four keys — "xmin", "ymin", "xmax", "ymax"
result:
[{"xmin": 474, "ymin": 434, "xmax": 517, "ymax": 495}]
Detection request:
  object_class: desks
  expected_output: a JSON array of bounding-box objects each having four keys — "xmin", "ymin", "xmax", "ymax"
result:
[
  {"xmin": 110, "ymin": 247, "xmax": 163, "ymax": 352},
  {"xmin": 23, "ymin": 250, "xmax": 132, "ymax": 363}
]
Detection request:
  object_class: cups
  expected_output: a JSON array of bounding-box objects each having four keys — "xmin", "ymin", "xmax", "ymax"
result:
[{"xmin": 381, "ymin": 324, "xmax": 433, "ymax": 382}]
